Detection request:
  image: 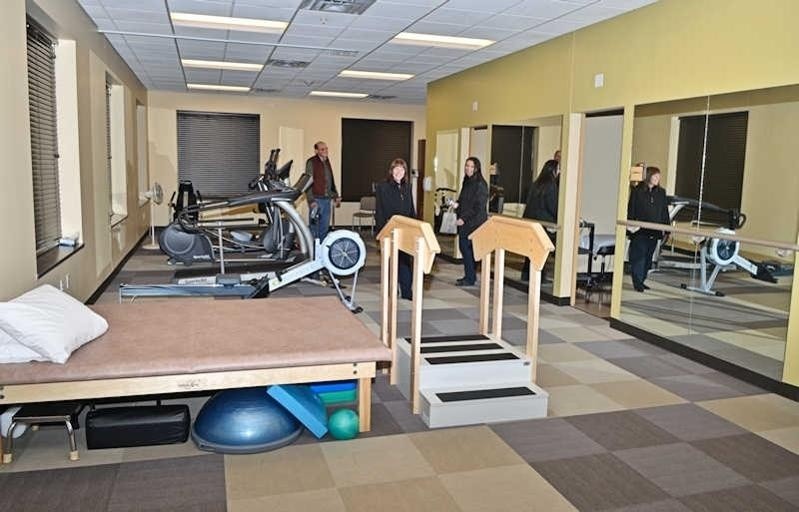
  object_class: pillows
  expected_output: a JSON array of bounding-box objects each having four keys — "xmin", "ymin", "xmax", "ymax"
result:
[
  {"xmin": 1, "ymin": 283, "xmax": 109, "ymax": 365},
  {"xmin": 0, "ymin": 329, "xmax": 52, "ymax": 363}
]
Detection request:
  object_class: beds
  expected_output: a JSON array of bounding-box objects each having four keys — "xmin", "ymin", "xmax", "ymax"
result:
[{"xmin": 0, "ymin": 295, "xmax": 392, "ymax": 432}]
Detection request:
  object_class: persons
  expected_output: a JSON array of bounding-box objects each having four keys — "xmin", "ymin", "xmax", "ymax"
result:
[
  {"xmin": 375, "ymin": 159, "xmax": 416, "ymax": 300},
  {"xmin": 452, "ymin": 158, "xmax": 488, "ymax": 286},
  {"xmin": 521, "ymin": 160, "xmax": 560, "ymax": 287},
  {"xmin": 628, "ymin": 165, "xmax": 671, "ymax": 292},
  {"xmin": 306, "ymin": 141, "xmax": 342, "ymax": 244},
  {"xmin": 553, "ymin": 150, "xmax": 561, "ymax": 185}
]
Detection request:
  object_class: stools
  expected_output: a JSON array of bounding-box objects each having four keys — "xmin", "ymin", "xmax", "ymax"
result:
[{"xmin": 3, "ymin": 400, "xmax": 96, "ymax": 465}]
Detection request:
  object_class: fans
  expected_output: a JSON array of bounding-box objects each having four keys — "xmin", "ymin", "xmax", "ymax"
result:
[{"xmin": 141, "ymin": 182, "xmax": 163, "ymax": 250}]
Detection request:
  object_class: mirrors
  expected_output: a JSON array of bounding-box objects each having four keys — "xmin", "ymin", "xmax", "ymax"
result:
[
  {"xmin": 619, "ymin": 83, "xmax": 799, "ymax": 381},
  {"xmin": 462, "ymin": 114, "xmax": 562, "ymax": 296}
]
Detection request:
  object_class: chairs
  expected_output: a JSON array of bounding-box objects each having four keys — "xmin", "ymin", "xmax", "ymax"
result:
[{"xmin": 351, "ymin": 197, "xmax": 377, "ymax": 241}]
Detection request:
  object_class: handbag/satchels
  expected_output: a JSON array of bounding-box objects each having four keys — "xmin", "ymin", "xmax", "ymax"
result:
[{"xmin": 439, "ymin": 212, "xmax": 458, "ymax": 234}]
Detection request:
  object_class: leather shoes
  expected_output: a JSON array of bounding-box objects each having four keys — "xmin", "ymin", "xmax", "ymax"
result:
[
  {"xmin": 455, "ymin": 277, "xmax": 475, "ymax": 286},
  {"xmin": 635, "ymin": 283, "xmax": 650, "ymax": 292}
]
